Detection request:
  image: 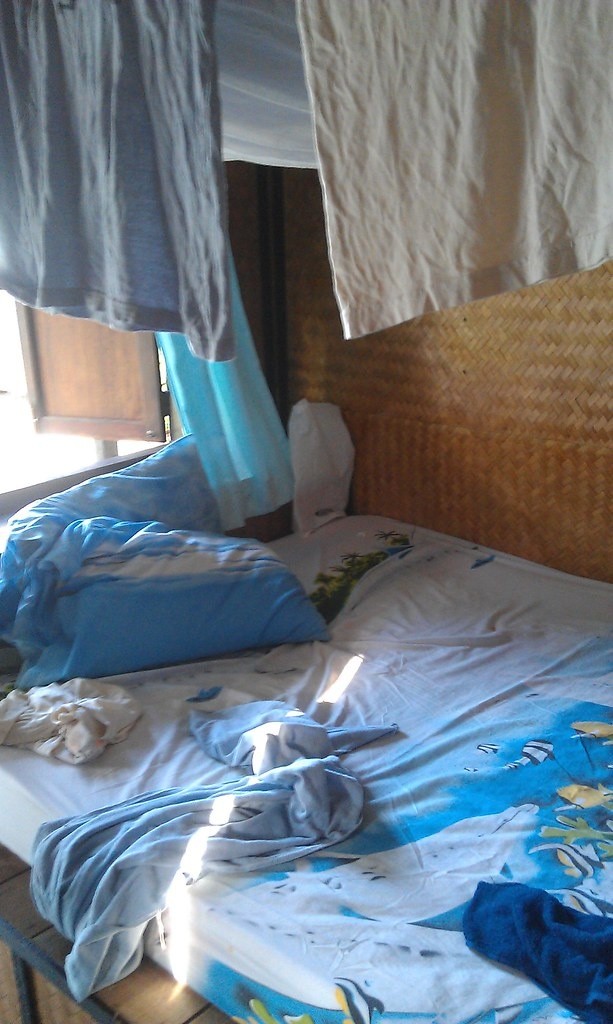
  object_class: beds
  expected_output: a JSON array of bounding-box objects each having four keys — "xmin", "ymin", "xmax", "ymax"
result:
[{"xmin": 0, "ymin": 514, "xmax": 613, "ymax": 1024}]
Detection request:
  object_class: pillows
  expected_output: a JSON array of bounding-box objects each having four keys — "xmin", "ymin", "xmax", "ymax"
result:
[{"xmin": 0, "ymin": 434, "xmax": 334, "ymax": 689}]
